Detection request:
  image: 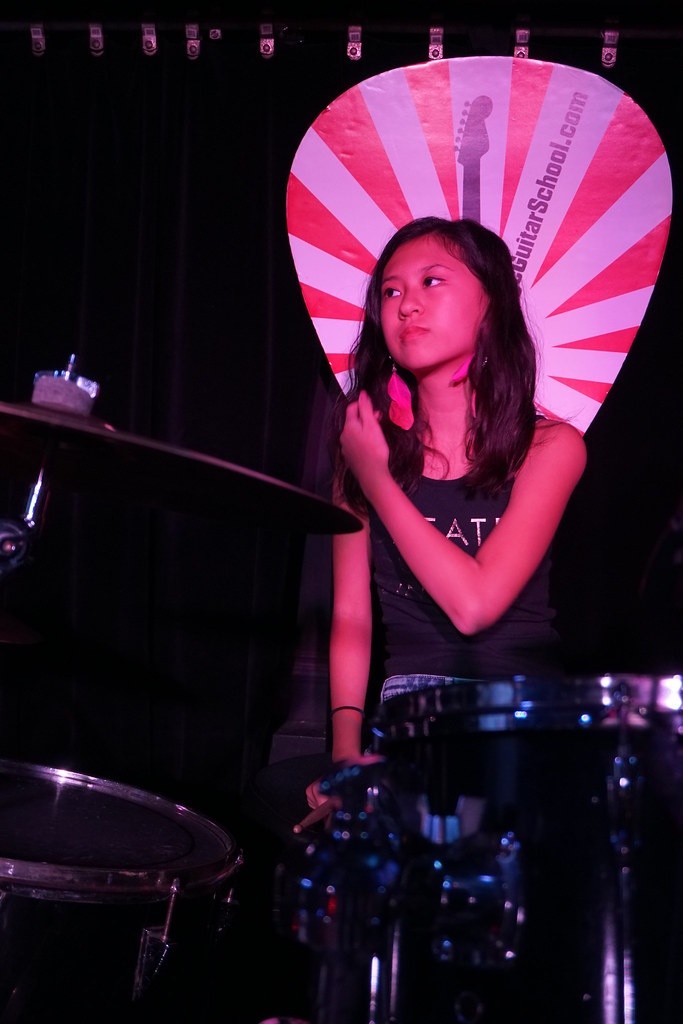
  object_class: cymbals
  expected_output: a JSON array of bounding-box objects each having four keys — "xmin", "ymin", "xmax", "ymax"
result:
[{"xmin": 0, "ymin": 399, "xmax": 364, "ymax": 534}]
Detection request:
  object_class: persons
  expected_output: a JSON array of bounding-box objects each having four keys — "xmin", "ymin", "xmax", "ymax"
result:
[{"xmin": 304, "ymin": 215, "xmax": 590, "ymax": 814}]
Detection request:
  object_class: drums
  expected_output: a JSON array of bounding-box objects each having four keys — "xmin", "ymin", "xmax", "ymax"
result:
[
  {"xmin": 0, "ymin": 758, "xmax": 236, "ymax": 1024},
  {"xmin": 324, "ymin": 670, "xmax": 683, "ymax": 1024}
]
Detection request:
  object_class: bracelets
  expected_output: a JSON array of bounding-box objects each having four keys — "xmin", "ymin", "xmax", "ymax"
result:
[{"xmin": 328, "ymin": 706, "xmax": 365, "ymax": 720}]
